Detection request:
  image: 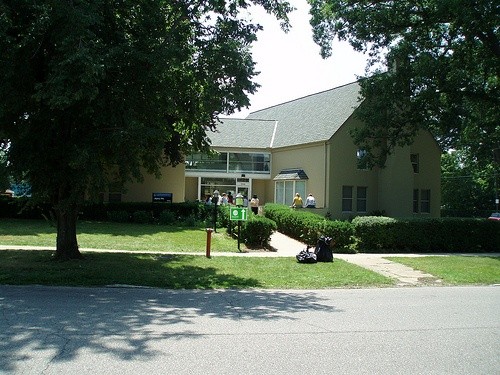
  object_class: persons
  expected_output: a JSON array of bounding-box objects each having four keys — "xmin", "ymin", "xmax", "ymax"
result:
[
  {"xmin": 472, "ymin": 207, "xmax": 478, "ymax": 218},
  {"xmin": 205, "ymin": 190, "xmax": 220, "ymax": 206},
  {"xmin": 305, "ymin": 193, "xmax": 316, "ymax": 208},
  {"xmin": 220, "ymin": 193, "xmax": 228, "ymax": 205},
  {"xmin": 227, "ymin": 192, "xmax": 233, "ymax": 204},
  {"xmin": 289, "ymin": 192, "xmax": 303, "ymax": 208},
  {"xmin": 234, "ymin": 193, "xmax": 259, "ymax": 215}
]
